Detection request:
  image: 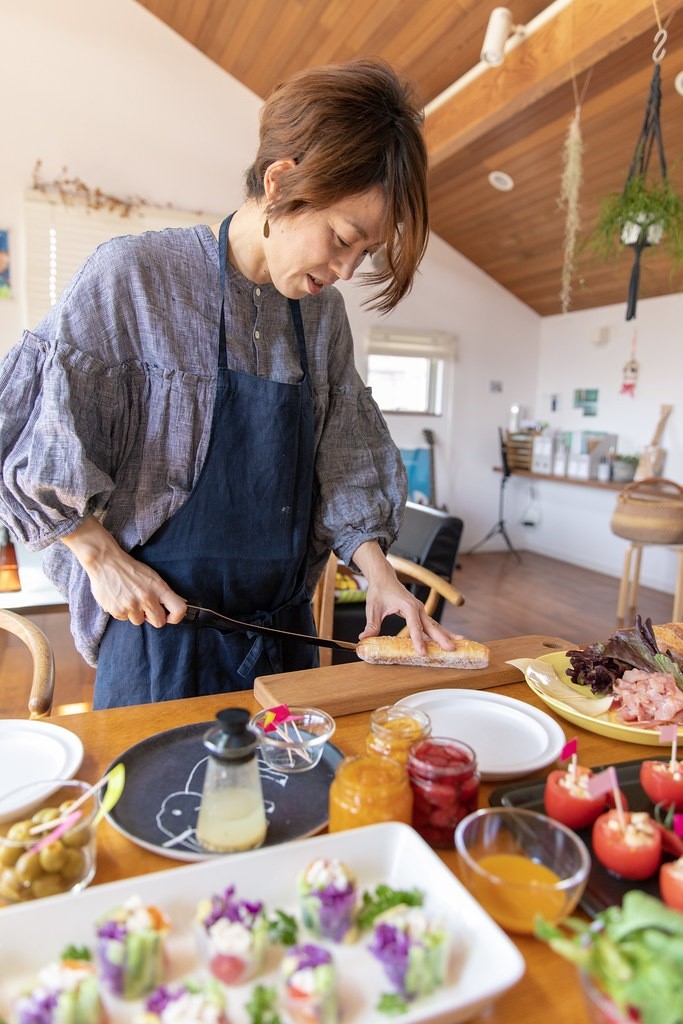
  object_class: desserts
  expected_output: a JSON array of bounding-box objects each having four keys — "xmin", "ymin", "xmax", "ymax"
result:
[{"xmin": 16, "ymin": 854, "xmax": 450, "ymax": 1024}]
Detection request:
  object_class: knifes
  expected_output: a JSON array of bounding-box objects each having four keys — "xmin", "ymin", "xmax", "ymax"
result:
[{"xmin": 98, "ymin": 605, "xmax": 358, "ymax": 651}]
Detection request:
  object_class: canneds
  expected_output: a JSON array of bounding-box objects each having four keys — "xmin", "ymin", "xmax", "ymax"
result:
[{"xmin": 328, "ymin": 705, "xmax": 481, "ymax": 851}]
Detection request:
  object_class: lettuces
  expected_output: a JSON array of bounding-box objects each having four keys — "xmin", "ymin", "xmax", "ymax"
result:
[
  {"xmin": 531, "ymin": 891, "xmax": 683, "ymax": 1024},
  {"xmin": 564, "ymin": 615, "xmax": 683, "ymax": 697}
]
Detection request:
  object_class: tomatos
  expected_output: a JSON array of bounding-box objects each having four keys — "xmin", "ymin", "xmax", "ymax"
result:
[{"xmin": 543, "ymin": 759, "xmax": 683, "ymax": 914}]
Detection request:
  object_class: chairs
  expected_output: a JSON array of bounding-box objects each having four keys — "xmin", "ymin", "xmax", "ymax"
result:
[{"xmin": 311, "ymin": 550, "xmax": 464, "ymax": 667}]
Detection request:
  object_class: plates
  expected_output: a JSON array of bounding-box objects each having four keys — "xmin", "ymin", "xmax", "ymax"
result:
[
  {"xmin": 96, "ymin": 721, "xmax": 346, "ymax": 861},
  {"xmin": 388, "ymin": 689, "xmax": 566, "ymax": 783},
  {"xmin": 0, "ymin": 719, "xmax": 84, "ymax": 819},
  {"xmin": 0, "ymin": 820, "xmax": 525, "ymax": 1024},
  {"xmin": 525, "ymin": 650, "xmax": 683, "ymax": 746}
]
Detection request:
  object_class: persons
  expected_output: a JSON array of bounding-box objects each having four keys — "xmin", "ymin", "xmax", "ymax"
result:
[{"xmin": 0, "ymin": 55, "xmax": 456, "ymax": 711}]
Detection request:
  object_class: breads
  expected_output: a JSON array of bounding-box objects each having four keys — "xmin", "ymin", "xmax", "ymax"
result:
[{"xmin": 356, "ymin": 635, "xmax": 490, "ymax": 670}]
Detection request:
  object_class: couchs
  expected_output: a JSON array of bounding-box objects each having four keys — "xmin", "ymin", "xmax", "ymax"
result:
[{"xmin": 332, "ymin": 501, "xmax": 464, "ymax": 664}]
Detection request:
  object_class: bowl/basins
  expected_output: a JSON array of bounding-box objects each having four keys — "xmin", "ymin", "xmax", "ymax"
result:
[
  {"xmin": 574, "ymin": 920, "xmax": 636, "ymax": 1024},
  {"xmin": 249, "ymin": 704, "xmax": 336, "ymax": 772},
  {"xmin": 454, "ymin": 807, "xmax": 589, "ymax": 935}
]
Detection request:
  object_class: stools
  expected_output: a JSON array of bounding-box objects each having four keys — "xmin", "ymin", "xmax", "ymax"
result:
[{"xmin": 617, "ymin": 540, "xmax": 682, "ymax": 623}]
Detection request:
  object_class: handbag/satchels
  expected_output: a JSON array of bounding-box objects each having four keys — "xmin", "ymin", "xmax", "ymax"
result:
[{"xmin": 610, "ymin": 479, "xmax": 683, "ymax": 544}]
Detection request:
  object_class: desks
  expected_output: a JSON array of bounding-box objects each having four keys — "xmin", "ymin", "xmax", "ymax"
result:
[{"xmin": 0, "ymin": 677, "xmax": 683, "ymax": 1024}]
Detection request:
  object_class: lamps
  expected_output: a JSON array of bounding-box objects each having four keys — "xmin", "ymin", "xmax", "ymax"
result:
[{"xmin": 480, "ymin": 7, "xmax": 523, "ymax": 65}]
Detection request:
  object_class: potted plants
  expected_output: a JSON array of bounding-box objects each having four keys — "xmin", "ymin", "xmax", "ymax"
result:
[{"xmin": 577, "ymin": 175, "xmax": 683, "ymax": 280}]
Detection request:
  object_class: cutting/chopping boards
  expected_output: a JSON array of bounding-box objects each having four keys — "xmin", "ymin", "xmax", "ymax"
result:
[{"xmin": 253, "ymin": 635, "xmax": 579, "ymax": 717}]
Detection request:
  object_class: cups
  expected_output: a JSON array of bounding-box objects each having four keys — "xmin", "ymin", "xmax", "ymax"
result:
[{"xmin": 0, "ymin": 780, "xmax": 101, "ymax": 905}]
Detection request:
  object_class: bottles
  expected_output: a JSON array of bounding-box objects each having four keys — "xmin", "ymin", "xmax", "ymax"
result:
[
  {"xmin": 365, "ymin": 706, "xmax": 432, "ymax": 768},
  {"xmin": 328, "ymin": 754, "xmax": 412, "ymax": 836},
  {"xmin": 196, "ymin": 706, "xmax": 266, "ymax": 851},
  {"xmin": 407, "ymin": 736, "xmax": 481, "ymax": 849}
]
